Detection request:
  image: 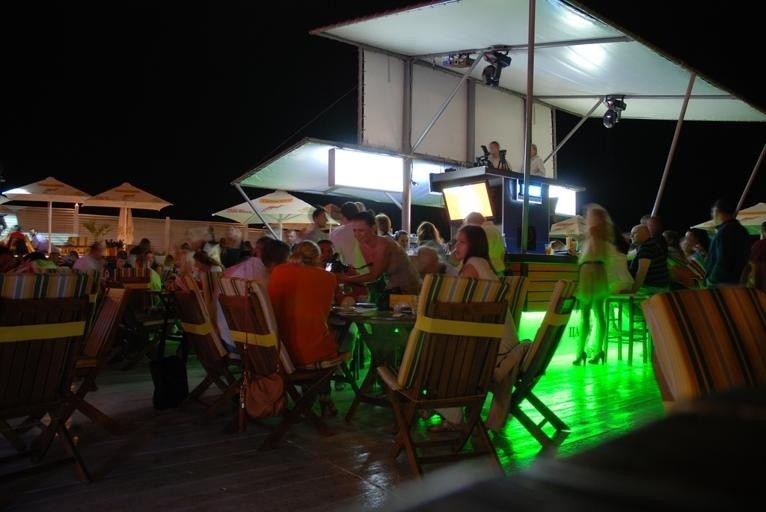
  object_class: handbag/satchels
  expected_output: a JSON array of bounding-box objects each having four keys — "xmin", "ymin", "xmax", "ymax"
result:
[{"xmin": 244, "ymin": 371, "xmax": 286, "ymax": 417}]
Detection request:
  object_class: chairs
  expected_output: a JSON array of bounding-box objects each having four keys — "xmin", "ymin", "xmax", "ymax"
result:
[
  {"xmin": 378, "ymin": 274, "xmax": 512, "ymax": 485},
  {"xmin": 2, "ymin": 271, "xmax": 92, "ymax": 485},
  {"xmin": 0, "ymin": 230, "xmax": 766, "ymax": 446}
]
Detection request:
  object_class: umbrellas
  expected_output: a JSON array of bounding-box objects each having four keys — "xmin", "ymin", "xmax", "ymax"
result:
[
  {"xmin": 82, "ymin": 182, "xmax": 174, "ymax": 251},
  {"xmin": 211, "ymin": 190, "xmax": 341, "ymax": 242},
  {"xmin": 1, "ymin": 176, "xmax": 92, "ymax": 257}
]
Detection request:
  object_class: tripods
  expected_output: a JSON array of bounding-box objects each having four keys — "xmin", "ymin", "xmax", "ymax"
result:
[{"xmin": 498, "ymin": 159, "xmax": 511, "ymax": 171}]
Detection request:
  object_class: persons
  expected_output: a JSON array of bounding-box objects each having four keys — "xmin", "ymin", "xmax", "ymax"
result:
[
  {"xmin": 1, "ymin": 225, "xmax": 222, "ymax": 339},
  {"xmin": 307, "ymin": 204, "xmax": 452, "ymax": 391},
  {"xmin": 548, "ymin": 204, "xmax": 766, "ymax": 368},
  {"xmin": 465, "ymin": 212, "xmax": 506, "ymax": 278},
  {"xmin": 2, "ymin": 203, "xmax": 528, "ymax": 432},
  {"xmin": 417, "ymin": 224, "xmax": 521, "ymax": 430},
  {"xmin": 217, "ymin": 230, "xmax": 340, "ymax": 417},
  {"xmin": 487, "ymin": 140, "xmax": 512, "ymax": 170},
  {"xmin": 520, "ymin": 143, "xmax": 546, "ymax": 177}
]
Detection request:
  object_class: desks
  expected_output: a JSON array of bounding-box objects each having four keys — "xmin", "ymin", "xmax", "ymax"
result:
[{"xmin": 429, "ymin": 165, "xmax": 590, "ymax": 253}]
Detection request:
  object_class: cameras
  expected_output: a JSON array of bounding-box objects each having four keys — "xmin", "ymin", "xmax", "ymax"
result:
[{"xmin": 325, "ymin": 261, "xmax": 339, "ymax": 272}]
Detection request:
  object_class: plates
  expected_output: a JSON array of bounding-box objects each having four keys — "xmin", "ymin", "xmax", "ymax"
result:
[{"xmin": 333, "ymin": 306, "xmax": 356, "ymax": 312}]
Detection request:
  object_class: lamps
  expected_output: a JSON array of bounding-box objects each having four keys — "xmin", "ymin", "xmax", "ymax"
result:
[
  {"xmin": 602, "ymin": 94, "xmax": 626, "ymax": 128},
  {"xmin": 479, "ymin": 50, "xmax": 510, "ymax": 88}
]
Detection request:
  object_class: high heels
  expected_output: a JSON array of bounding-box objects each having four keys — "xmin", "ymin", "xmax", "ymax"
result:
[
  {"xmin": 589, "ymin": 351, "xmax": 604, "ymax": 364},
  {"xmin": 573, "ymin": 352, "xmax": 586, "ymax": 366}
]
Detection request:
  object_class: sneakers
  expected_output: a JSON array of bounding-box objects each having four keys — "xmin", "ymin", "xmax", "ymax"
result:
[{"xmin": 320, "ymin": 379, "xmax": 345, "ymax": 420}]
